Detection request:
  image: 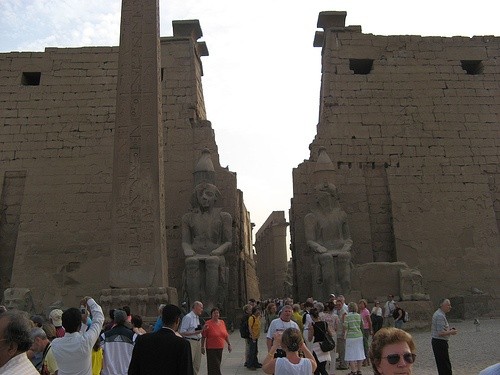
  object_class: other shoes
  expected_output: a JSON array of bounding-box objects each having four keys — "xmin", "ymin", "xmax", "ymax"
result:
[
  {"xmin": 244, "ymin": 363, "xmax": 248, "ymax": 366},
  {"xmin": 259, "ymin": 363, "xmax": 263, "ymax": 366},
  {"xmin": 256, "ymin": 365, "xmax": 262, "ymax": 368},
  {"xmin": 336, "ymin": 367, "xmax": 349, "ymax": 370}
]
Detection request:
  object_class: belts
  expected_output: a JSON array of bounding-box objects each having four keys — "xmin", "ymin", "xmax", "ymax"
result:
[{"xmin": 184, "ymin": 337, "xmax": 200, "ymax": 341}]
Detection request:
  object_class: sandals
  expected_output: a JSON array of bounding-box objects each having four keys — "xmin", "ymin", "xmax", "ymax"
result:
[
  {"xmin": 348, "ymin": 371, "xmax": 357, "ymax": 375},
  {"xmin": 357, "ymin": 371, "xmax": 363, "ymax": 375}
]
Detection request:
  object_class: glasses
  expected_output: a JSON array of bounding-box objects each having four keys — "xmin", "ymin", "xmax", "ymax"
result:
[{"xmin": 377, "ymin": 353, "xmax": 416, "ymax": 365}]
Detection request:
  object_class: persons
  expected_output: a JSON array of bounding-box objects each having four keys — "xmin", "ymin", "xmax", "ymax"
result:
[
  {"xmin": 308, "ymin": 308, "xmax": 334, "ymax": 375},
  {"xmin": 240, "ymin": 297, "xmax": 295, "ymax": 369},
  {"xmin": 431, "ymin": 299, "xmax": 457, "ymax": 375},
  {"xmin": 179, "ymin": 301, "xmax": 205, "ymax": 375},
  {"xmin": 369, "ymin": 327, "xmax": 417, "ymax": 375},
  {"xmin": 358, "ymin": 294, "xmax": 404, "ymax": 367},
  {"xmin": 201, "ymin": 307, "xmax": 232, "ymax": 375},
  {"xmin": 292, "ymin": 294, "xmax": 350, "ymax": 375},
  {"xmin": 126, "ymin": 304, "xmax": 194, "ymax": 375},
  {"xmin": 304, "ymin": 183, "xmax": 354, "ymax": 297},
  {"xmin": 0, "ymin": 310, "xmax": 40, "ymax": 375},
  {"xmin": 0, "ymin": 299, "xmax": 147, "ymax": 375},
  {"xmin": 181, "ymin": 182, "xmax": 232, "ymax": 314},
  {"xmin": 262, "ymin": 328, "xmax": 317, "ymax": 375},
  {"xmin": 152, "ymin": 304, "xmax": 166, "ymax": 331},
  {"xmin": 342, "ymin": 302, "xmax": 367, "ymax": 375},
  {"xmin": 51, "ymin": 297, "xmax": 105, "ymax": 375}
]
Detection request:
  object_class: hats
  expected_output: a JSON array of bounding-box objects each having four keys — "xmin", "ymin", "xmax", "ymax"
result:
[
  {"xmin": 330, "ymin": 294, "xmax": 335, "ymax": 297},
  {"xmin": 114, "ymin": 310, "xmax": 127, "ymax": 322},
  {"xmin": 49, "ymin": 309, "xmax": 63, "ymax": 326}
]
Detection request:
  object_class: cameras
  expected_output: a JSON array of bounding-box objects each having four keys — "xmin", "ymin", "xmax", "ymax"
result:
[{"xmin": 81, "ymin": 305, "xmax": 87, "ymax": 314}]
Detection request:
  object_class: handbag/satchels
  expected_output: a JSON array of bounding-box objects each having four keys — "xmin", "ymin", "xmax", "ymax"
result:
[{"xmin": 319, "ymin": 334, "xmax": 335, "ymax": 352}]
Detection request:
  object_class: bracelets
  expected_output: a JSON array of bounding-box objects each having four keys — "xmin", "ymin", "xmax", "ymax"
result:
[{"xmin": 228, "ymin": 343, "xmax": 231, "ymax": 345}]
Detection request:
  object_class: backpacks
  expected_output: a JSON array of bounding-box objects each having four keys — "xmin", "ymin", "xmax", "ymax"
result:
[
  {"xmin": 239, "ymin": 315, "xmax": 255, "ymax": 338},
  {"xmin": 397, "ymin": 308, "xmax": 408, "ymax": 323}
]
